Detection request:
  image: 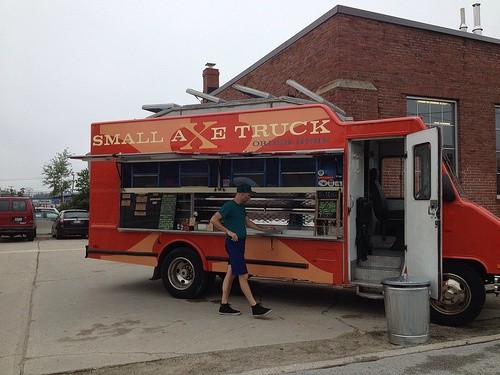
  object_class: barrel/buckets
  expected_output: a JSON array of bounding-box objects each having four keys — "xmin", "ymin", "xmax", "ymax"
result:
[{"xmin": 383, "ymin": 275, "xmax": 431, "ymax": 345}]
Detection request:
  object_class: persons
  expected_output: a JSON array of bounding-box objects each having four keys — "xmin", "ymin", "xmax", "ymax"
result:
[{"xmin": 210, "ymin": 185, "xmax": 276, "ymax": 317}]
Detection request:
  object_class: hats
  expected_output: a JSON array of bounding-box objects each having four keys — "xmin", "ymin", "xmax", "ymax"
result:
[{"xmin": 237, "ymin": 184, "xmax": 256, "ymax": 193}]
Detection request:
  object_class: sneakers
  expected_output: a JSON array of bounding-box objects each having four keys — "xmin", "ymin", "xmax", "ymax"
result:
[
  {"xmin": 219, "ymin": 304, "xmax": 241, "ymax": 314},
  {"xmin": 252, "ymin": 304, "xmax": 272, "ymax": 316}
]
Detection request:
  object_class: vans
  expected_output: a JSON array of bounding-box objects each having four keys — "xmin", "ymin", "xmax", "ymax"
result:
[{"xmin": 0, "ymin": 196, "xmax": 39, "ymax": 241}]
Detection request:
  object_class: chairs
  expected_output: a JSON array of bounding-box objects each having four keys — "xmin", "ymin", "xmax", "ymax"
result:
[{"xmin": 369, "ymin": 168, "xmax": 404, "ymax": 242}]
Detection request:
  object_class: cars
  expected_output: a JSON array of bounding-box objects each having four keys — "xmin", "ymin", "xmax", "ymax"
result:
[{"xmin": 51, "ymin": 208, "xmax": 89, "ymax": 240}]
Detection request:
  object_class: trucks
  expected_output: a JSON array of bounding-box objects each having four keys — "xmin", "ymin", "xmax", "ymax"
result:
[{"xmin": 69, "ymin": 77, "xmax": 500, "ymax": 328}]
